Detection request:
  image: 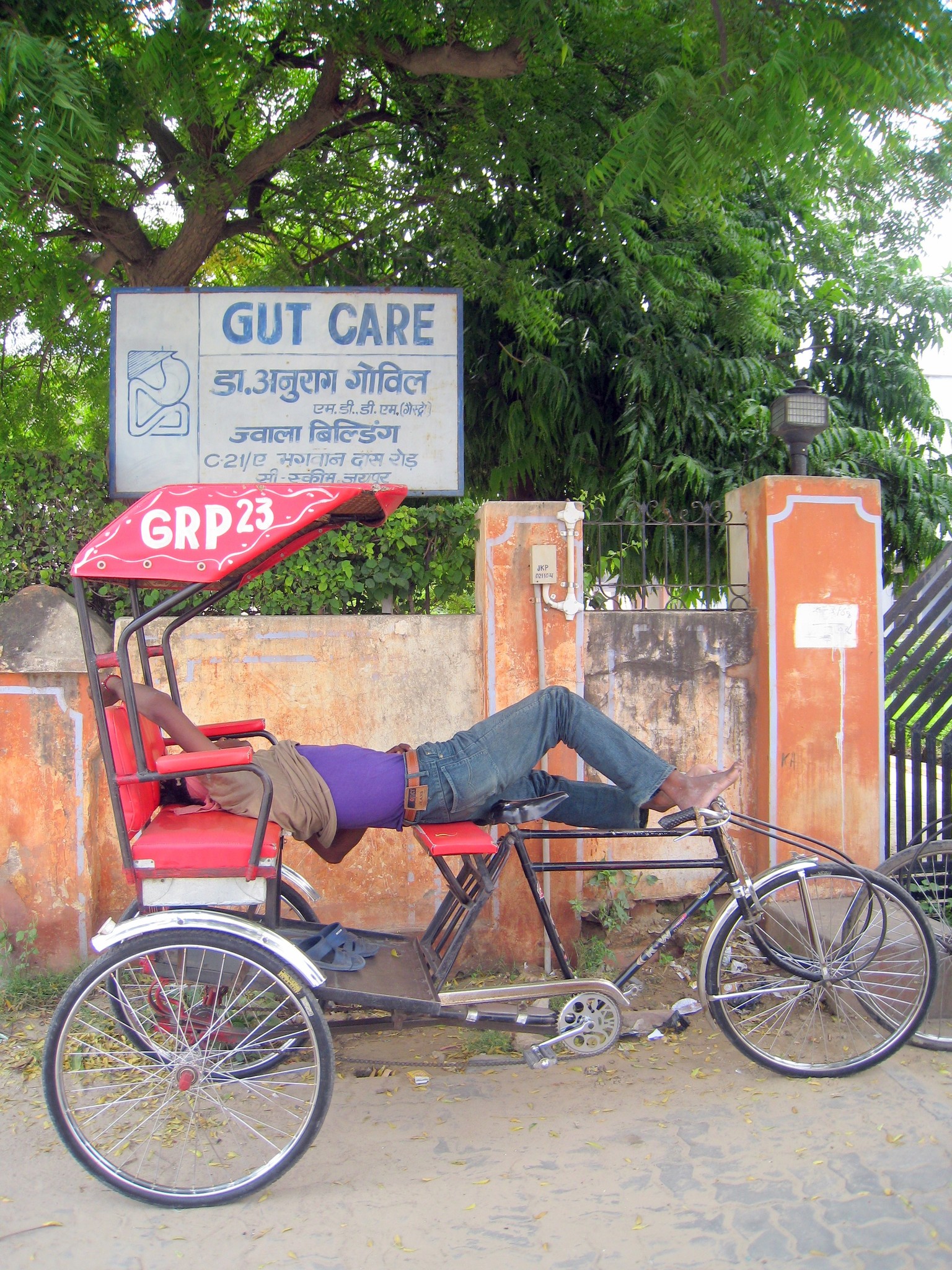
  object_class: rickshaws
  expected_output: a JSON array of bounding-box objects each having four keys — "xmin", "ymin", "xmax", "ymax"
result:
[{"xmin": 39, "ymin": 488, "xmax": 933, "ymax": 1211}]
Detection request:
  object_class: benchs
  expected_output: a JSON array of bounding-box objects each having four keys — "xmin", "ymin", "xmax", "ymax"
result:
[{"xmin": 99, "ymin": 697, "xmax": 285, "ymax": 923}]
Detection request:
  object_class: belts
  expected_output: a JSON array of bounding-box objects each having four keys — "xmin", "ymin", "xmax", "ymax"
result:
[{"xmin": 402, "ymin": 750, "xmax": 420, "ymax": 826}]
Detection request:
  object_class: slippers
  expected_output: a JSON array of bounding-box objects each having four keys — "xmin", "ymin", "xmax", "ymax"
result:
[
  {"xmin": 291, "ymin": 922, "xmax": 379, "ymax": 957},
  {"xmin": 298, "ymin": 934, "xmax": 365, "ymax": 971}
]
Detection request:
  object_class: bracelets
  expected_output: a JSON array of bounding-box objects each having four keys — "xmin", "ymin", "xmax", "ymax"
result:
[{"xmin": 101, "ymin": 675, "xmax": 122, "ymax": 693}]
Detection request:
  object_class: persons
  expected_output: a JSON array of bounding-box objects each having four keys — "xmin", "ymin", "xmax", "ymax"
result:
[{"xmin": 85, "ymin": 669, "xmax": 743, "ymax": 869}]
